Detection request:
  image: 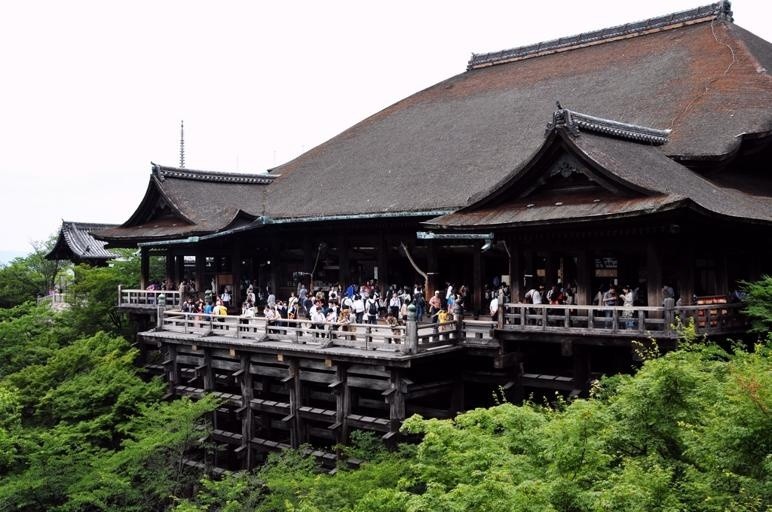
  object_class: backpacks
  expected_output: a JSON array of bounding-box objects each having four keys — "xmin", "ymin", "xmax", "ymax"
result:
[{"xmin": 368, "ymin": 300, "xmax": 376, "ymax": 315}]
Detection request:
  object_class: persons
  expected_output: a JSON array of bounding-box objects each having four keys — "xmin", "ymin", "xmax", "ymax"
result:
[
  {"xmin": 147, "ymin": 279, "xmax": 196, "ymax": 305},
  {"xmin": 184, "ymin": 288, "xmax": 231, "ymax": 329},
  {"xmin": 490, "ymin": 282, "xmax": 511, "ymax": 322},
  {"xmin": 602, "ymin": 285, "xmax": 634, "ymax": 331},
  {"xmin": 241, "ymin": 281, "xmax": 469, "ymax": 342},
  {"xmin": 723, "ymin": 290, "xmax": 746, "ymax": 326},
  {"xmin": 49, "ymin": 286, "xmax": 59, "ymax": 296},
  {"xmin": 525, "ymin": 283, "xmax": 574, "ymax": 326},
  {"xmin": 662, "ymin": 286, "xmax": 674, "ymax": 317}
]
